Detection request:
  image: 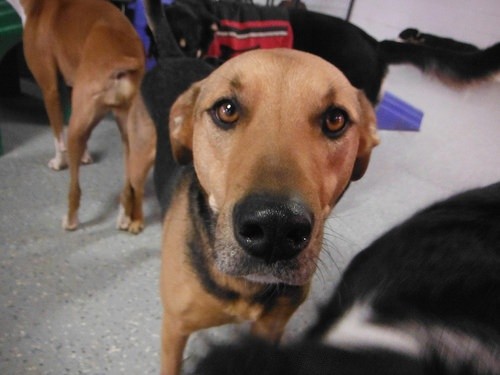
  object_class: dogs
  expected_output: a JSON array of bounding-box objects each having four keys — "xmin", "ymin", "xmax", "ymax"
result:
[
  {"xmin": 180, "ymin": 175, "xmax": 500, "ymax": 375},
  {"xmin": 114, "ymin": 0, "xmax": 382, "ymax": 375},
  {"xmin": 143, "ymin": 0, "xmax": 500, "ymax": 110},
  {"xmin": 5, "ymin": 1, "xmax": 148, "ymax": 233}
]
